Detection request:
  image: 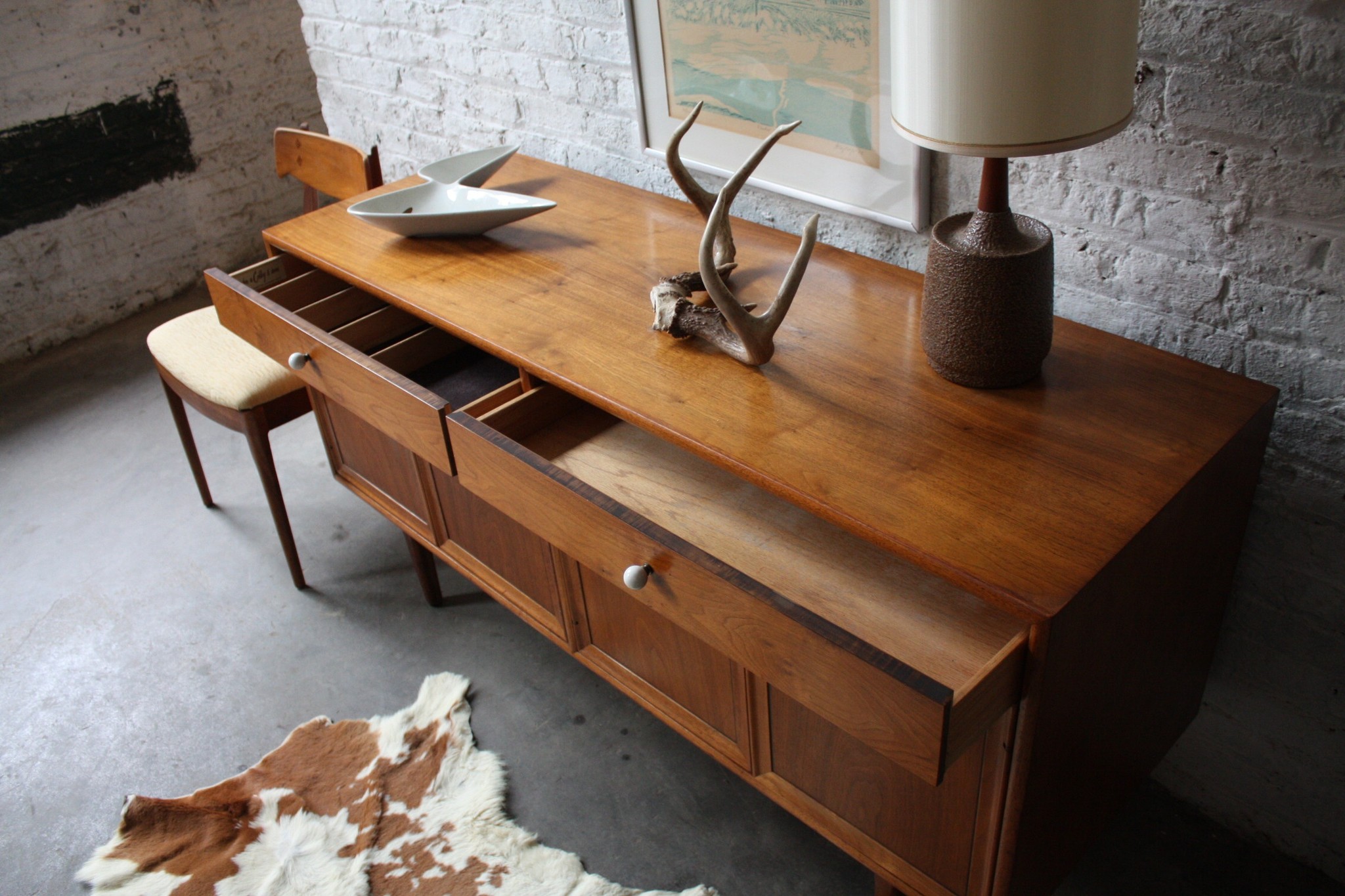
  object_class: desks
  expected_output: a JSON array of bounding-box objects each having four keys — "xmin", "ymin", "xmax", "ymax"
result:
[{"xmin": 203, "ymin": 152, "xmax": 1280, "ymax": 896}]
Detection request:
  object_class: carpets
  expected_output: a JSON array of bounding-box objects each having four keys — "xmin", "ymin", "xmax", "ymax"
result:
[{"xmin": 78, "ymin": 670, "xmax": 718, "ymax": 896}]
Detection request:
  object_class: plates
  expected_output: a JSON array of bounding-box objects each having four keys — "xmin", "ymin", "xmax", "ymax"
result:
[{"xmin": 347, "ymin": 145, "xmax": 557, "ymax": 239}]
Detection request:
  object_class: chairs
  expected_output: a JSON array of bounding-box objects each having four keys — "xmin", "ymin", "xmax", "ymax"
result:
[{"xmin": 145, "ymin": 121, "xmax": 388, "ymax": 589}]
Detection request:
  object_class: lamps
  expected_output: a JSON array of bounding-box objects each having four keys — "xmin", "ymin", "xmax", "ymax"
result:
[{"xmin": 890, "ymin": 1, "xmax": 1145, "ymax": 391}]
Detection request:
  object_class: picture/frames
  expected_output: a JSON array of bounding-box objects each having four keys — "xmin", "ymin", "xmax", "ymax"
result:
[{"xmin": 616, "ymin": 0, "xmax": 933, "ymax": 232}]
